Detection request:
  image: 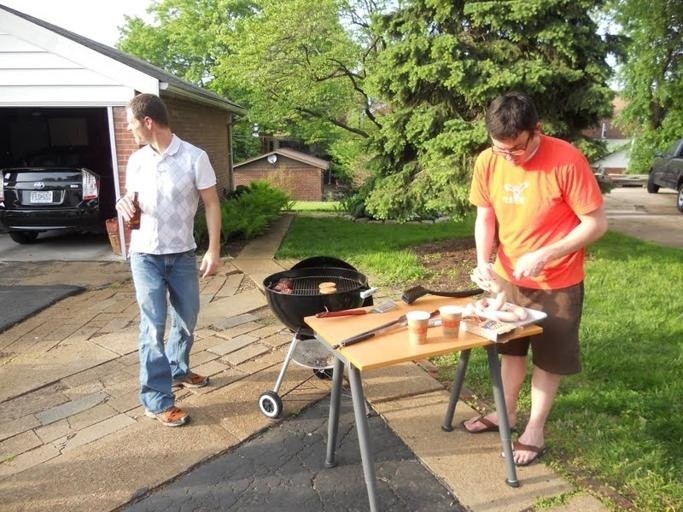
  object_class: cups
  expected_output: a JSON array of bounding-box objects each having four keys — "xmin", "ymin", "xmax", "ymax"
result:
[
  {"xmin": 438, "ymin": 306, "xmax": 462, "ymax": 337},
  {"xmin": 405, "ymin": 311, "xmax": 431, "ymax": 344}
]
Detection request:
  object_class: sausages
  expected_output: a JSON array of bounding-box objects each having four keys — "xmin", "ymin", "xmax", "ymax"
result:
[
  {"xmin": 502, "ymin": 303, "xmax": 528, "ymax": 321},
  {"xmin": 484, "ymin": 290, "xmax": 506, "ymax": 311},
  {"xmin": 483, "ymin": 311, "xmax": 519, "ymax": 322}
]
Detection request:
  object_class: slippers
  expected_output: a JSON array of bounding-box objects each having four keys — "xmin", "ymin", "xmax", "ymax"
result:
[
  {"xmin": 458, "ymin": 412, "xmax": 519, "ymax": 435},
  {"xmin": 499, "ymin": 438, "xmax": 551, "ymax": 467}
]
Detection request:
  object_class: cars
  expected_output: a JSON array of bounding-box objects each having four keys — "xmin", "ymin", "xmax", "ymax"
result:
[
  {"xmin": 0, "ymin": 141, "xmax": 112, "ymax": 244},
  {"xmin": 649, "ymin": 137, "xmax": 683, "ymax": 213}
]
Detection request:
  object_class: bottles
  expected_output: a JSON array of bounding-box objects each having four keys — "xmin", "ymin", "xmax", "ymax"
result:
[{"xmin": 128, "ymin": 191, "xmax": 139, "ymax": 230}]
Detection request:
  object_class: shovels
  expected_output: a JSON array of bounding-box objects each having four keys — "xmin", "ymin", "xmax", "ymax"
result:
[
  {"xmin": 401, "ymin": 285, "xmax": 484, "ymax": 304},
  {"xmin": 316, "ymin": 300, "xmax": 398, "ymax": 318}
]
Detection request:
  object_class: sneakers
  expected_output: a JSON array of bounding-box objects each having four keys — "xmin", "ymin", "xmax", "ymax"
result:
[
  {"xmin": 170, "ymin": 369, "xmax": 211, "ymax": 391},
  {"xmin": 144, "ymin": 402, "xmax": 193, "ymax": 429}
]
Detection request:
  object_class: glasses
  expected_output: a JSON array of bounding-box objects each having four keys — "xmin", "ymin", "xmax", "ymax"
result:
[{"xmin": 489, "ymin": 133, "xmax": 533, "ymax": 158}]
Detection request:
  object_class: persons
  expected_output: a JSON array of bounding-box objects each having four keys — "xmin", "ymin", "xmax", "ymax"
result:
[
  {"xmin": 459, "ymin": 89, "xmax": 609, "ymax": 467},
  {"xmin": 113, "ymin": 92, "xmax": 222, "ymax": 427}
]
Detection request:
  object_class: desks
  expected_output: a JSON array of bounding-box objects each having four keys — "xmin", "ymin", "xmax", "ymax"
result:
[{"xmin": 297, "ymin": 292, "xmax": 545, "ymax": 511}]
